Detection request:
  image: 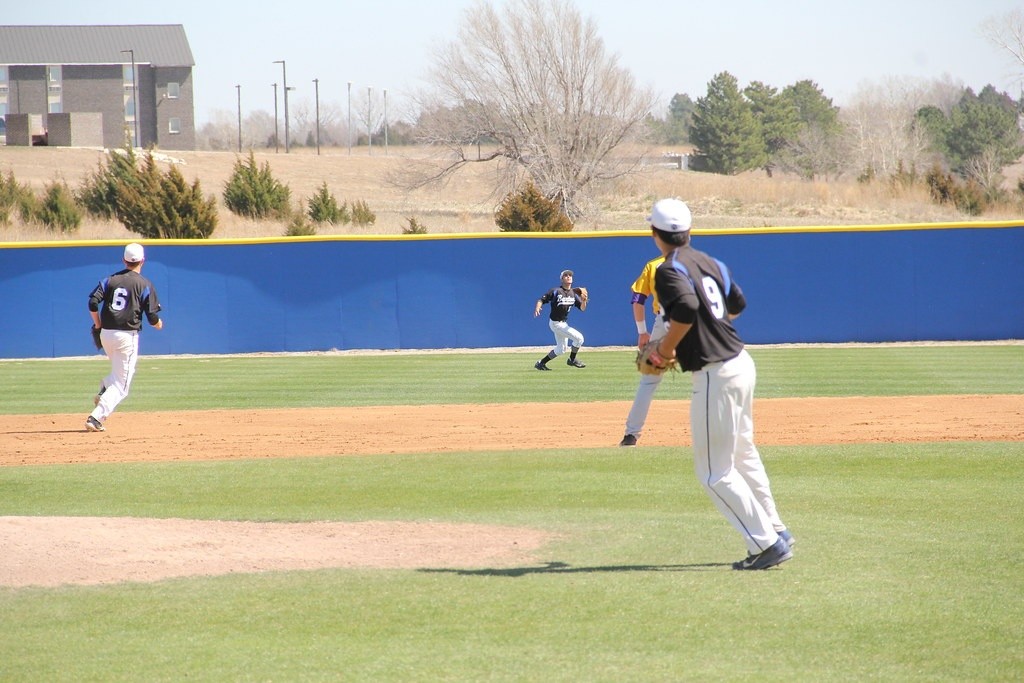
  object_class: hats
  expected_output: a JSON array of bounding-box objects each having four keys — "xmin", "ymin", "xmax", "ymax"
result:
[
  {"xmin": 124, "ymin": 243, "xmax": 144, "ymax": 262},
  {"xmin": 646, "ymin": 198, "xmax": 691, "ymax": 233},
  {"xmin": 560, "ymin": 269, "xmax": 574, "ymax": 280}
]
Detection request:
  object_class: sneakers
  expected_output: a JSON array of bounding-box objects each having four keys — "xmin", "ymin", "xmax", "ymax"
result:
[
  {"xmin": 95, "ymin": 394, "xmax": 102, "ymax": 406},
  {"xmin": 778, "ymin": 529, "xmax": 796, "ymax": 547},
  {"xmin": 84, "ymin": 417, "xmax": 106, "ymax": 432},
  {"xmin": 731, "ymin": 536, "xmax": 793, "ymax": 570}
]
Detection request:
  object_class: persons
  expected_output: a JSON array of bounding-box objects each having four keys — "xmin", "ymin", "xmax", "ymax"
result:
[
  {"xmin": 534, "ymin": 270, "xmax": 588, "ymax": 371},
  {"xmin": 620, "ymin": 256, "xmax": 667, "ymax": 446},
  {"xmin": 85, "ymin": 244, "xmax": 162, "ymax": 431},
  {"xmin": 636, "ymin": 198, "xmax": 796, "ymax": 570}
]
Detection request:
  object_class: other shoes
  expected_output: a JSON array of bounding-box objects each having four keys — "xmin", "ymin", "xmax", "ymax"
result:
[
  {"xmin": 620, "ymin": 434, "xmax": 637, "ymax": 446},
  {"xmin": 534, "ymin": 361, "xmax": 552, "ymax": 371},
  {"xmin": 567, "ymin": 359, "xmax": 586, "ymax": 368}
]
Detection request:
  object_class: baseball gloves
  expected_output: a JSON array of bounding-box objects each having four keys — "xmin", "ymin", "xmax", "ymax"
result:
[
  {"xmin": 573, "ymin": 287, "xmax": 588, "ymax": 301},
  {"xmin": 633, "ymin": 335, "xmax": 680, "ymax": 382},
  {"xmin": 90, "ymin": 324, "xmax": 103, "ymax": 352}
]
